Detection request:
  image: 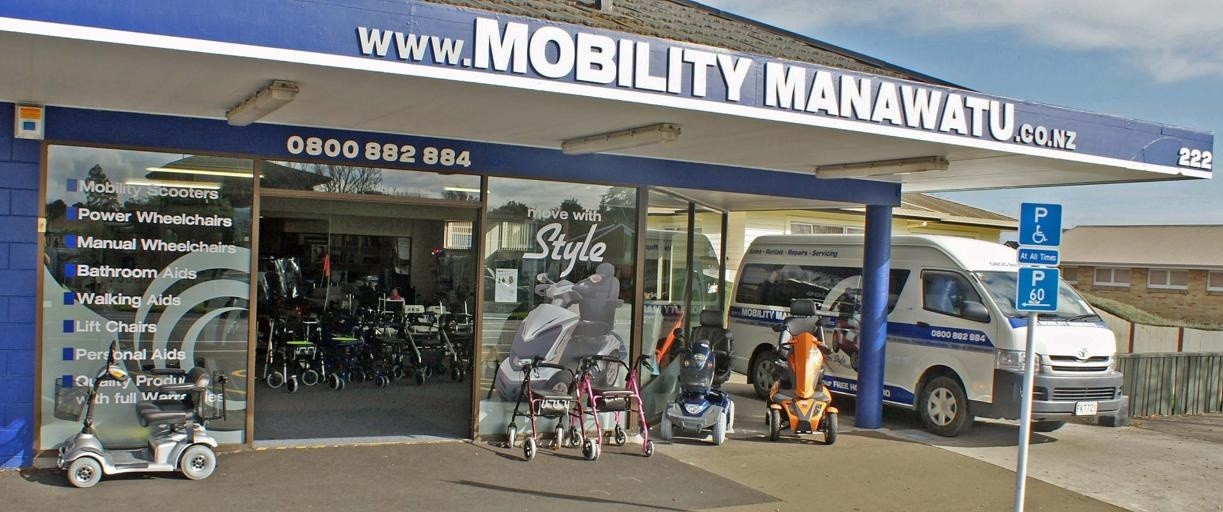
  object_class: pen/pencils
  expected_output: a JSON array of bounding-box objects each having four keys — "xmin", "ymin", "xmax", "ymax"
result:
[{"xmin": 727, "ymin": 233, "xmax": 1124, "ymax": 438}]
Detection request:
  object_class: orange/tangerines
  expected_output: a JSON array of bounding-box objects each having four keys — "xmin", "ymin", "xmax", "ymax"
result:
[
  {"xmin": 505, "ymin": 354, "xmax": 655, "ymax": 462},
  {"xmin": 262, "ymin": 298, "xmax": 474, "ymax": 394}
]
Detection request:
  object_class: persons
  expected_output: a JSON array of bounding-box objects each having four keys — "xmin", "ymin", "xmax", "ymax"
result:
[
  {"xmin": 558, "ymin": 262, "xmax": 621, "ymax": 369},
  {"xmin": 385, "ymin": 285, "xmax": 406, "ymax": 309},
  {"xmin": 836, "ymin": 287, "xmax": 862, "ymax": 335}
]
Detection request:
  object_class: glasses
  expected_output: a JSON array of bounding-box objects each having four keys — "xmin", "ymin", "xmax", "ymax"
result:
[
  {"xmin": 561, "ymin": 124, "xmax": 681, "ymax": 155},
  {"xmin": 124, "ymin": 164, "xmax": 265, "ymax": 190},
  {"xmin": 225, "ymin": 80, "xmax": 300, "ymax": 127},
  {"xmin": 814, "ymin": 157, "xmax": 950, "ymax": 179}
]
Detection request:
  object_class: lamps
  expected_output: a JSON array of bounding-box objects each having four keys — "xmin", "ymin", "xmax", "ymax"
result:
[
  {"xmin": 136, "ymin": 368, "xmax": 210, "ymax": 427},
  {"xmin": 678, "ymin": 309, "xmax": 733, "ymax": 385},
  {"xmin": 774, "ymin": 299, "xmax": 832, "ymax": 378}
]
